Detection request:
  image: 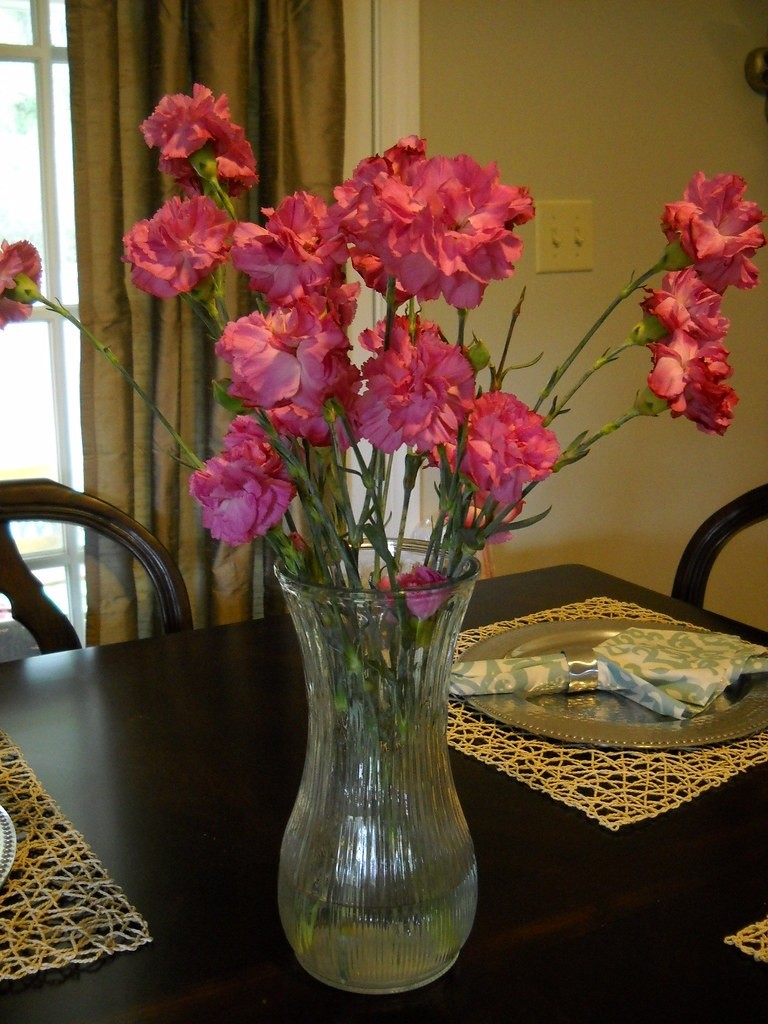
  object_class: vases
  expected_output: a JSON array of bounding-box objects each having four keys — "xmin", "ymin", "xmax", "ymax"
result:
[{"xmin": 269, "ymin": 535, "xmax": 480, "ymax": 994}]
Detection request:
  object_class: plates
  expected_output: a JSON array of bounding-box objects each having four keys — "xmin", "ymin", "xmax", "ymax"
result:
[
  {"xmin": 456, "ymin": 619, "xmax": 768, "ymax": 749},
  {"xmin": 0, "ymin": 806, "xmax": 16, "ymax": 888}
]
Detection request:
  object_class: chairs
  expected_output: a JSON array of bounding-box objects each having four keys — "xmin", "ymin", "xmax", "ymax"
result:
[
  {"xmin": 0, "ymin": 478, "xmax": 192, "ymax": 667},
  {"xmin": 669, "ymin": 483, "xmax": 768, "ymax": 628}
]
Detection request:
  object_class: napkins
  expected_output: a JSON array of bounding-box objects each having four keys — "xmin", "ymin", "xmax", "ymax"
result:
[{"xmin": 445, "ymin": 626, "xmax": 764, "ymax": 719}]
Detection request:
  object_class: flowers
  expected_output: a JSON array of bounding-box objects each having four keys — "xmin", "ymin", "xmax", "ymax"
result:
[{"xmin": 0, "ymin": 83, "xmax": 768, "ymax": 980}]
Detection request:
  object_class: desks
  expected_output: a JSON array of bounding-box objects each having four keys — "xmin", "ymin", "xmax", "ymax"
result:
[{"xmin": 1, "ymin": 562, "xmax": 768, "ymax": 1024}]
